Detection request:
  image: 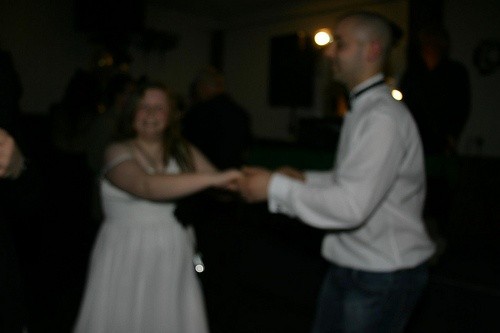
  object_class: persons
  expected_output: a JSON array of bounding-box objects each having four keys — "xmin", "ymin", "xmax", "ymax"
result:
[
  {"xmin": 0, "ymin": 60, "xmax": 23, "ymax": 323},
  {"xmin": 232, "ymin": 10, "xmax": 439, "ymax": 333},
  {"xmin": 180, "ymin": 67, "xmax": 251, "ymax": 272},
  {"xmin": 70, "ymin": 77, "xmax": 245, "ymax": 333}
]
